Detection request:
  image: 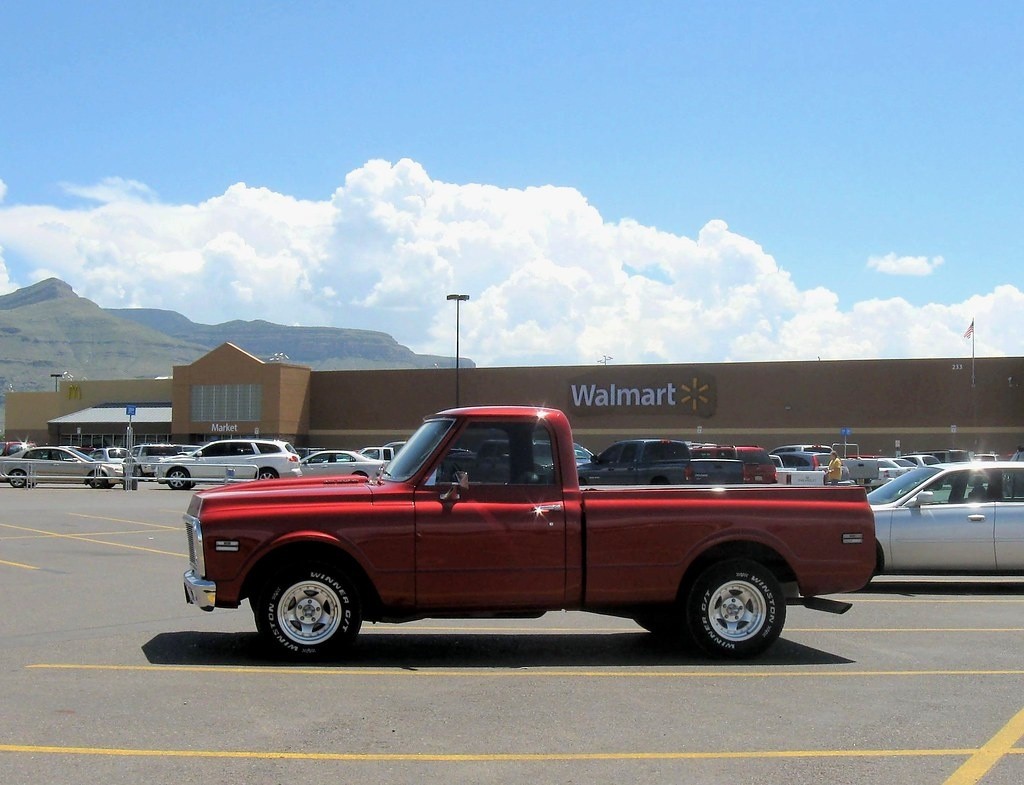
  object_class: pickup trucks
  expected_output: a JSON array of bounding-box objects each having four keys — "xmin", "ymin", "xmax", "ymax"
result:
[{"xmin": 181, "ymin": 405, "xmax": 878, "ymax": 659}]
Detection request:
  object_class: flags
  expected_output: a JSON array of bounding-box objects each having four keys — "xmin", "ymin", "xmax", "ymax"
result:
[{"xmin": 962, "ymin": 321, "xmax": 974, "ymax": 340}]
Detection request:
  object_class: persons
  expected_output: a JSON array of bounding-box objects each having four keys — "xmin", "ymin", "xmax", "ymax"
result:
[{"xmin": 826, "ymin": 451, "xmax": 842, "ymax": 486}]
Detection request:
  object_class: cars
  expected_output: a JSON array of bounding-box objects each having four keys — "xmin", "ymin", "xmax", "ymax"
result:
[
  {"xmin": 0, "ymin": 433, "xmax": 1023, "ymax": 490},
  {"xmin": 857, "ymin": 460, "xmax": 1023, "ymax": 585}
]
